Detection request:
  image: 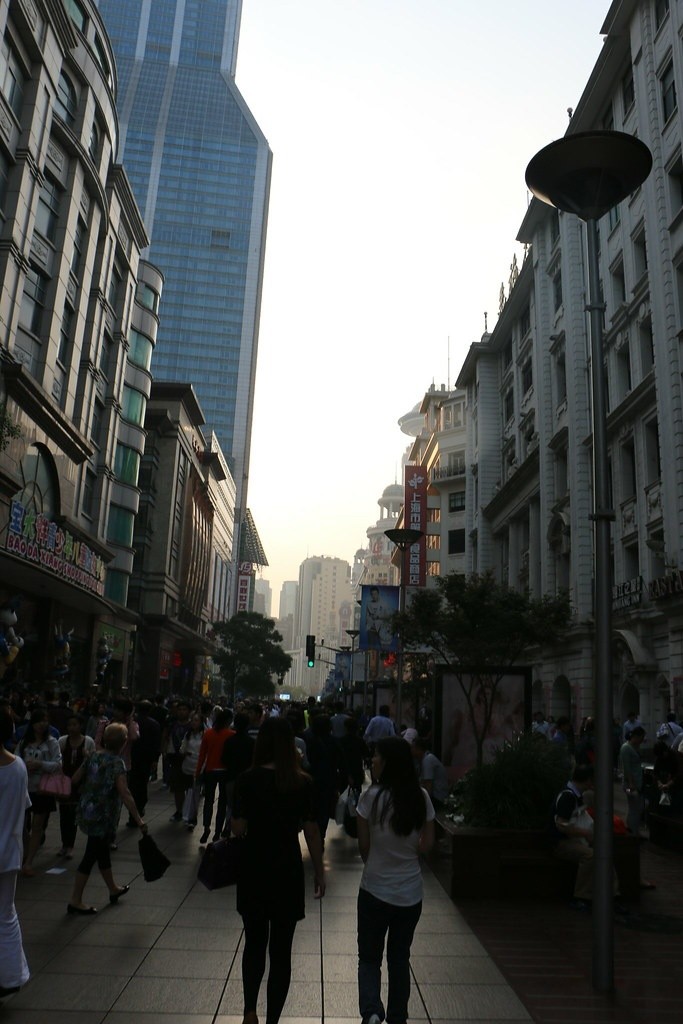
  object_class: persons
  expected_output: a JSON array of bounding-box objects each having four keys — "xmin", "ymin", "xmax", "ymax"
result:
[
  {"xmin": 229, "ymin": 726, "xmax": 326, "ymax": 1024},
  {"xmin": 531, "ymin": 711, "xmax": 683, "ymax": 889},
  {"xmin": 356, "ymin": 736, "xmax": 435, "ymax": 1024},
  {"xmin": 553, "ymin": 789, "xmax": 633, "ymax": 924},
  {"xmin": 1, "ymin": 679, "xmax": 451, "ymax": 914},
  {"xmin": 363, "ymin": 587, "xmax": 396, "ymax": 649},
  {"xmin": 338, "ymin": 654, "xmax": 346, "ymax": 672},
  {"xmin": 0, "ymin": 710, "xmax": 33, "ymax": 997}
]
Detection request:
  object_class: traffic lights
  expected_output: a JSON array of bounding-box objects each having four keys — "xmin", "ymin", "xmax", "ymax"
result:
[
  {"xmin": 306, "ymin": 635, "xmax": 315, "ymax": 657},
  {"xmin": 337, "ymin": 686, "xmax": 343, "ymax": 693},
  {"xmin": 308, "ymin": 656, "xmax": 314, "ymax": 667}
]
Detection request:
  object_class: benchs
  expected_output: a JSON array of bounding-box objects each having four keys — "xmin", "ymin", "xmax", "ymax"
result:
[{"xmin": 495, "ymin": 849, "xmax": 567, "ymax": 867}]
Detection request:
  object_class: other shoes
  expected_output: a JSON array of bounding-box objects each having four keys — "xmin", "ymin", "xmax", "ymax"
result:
[
  {"xmin": 212, "ymin": 832, "xmax": 220, "ymax": 842},
  {"xmin": 109, "ymin": 885, "xmax": 130, "ymax": 906},
  {"xmin": 126, "ymin": 820, "xmax": 139, "ymax": 828},
  {"xmin": 200, "ymin": 829, "xmax": 211, "ymax": 843},
  {"xmin": 67, "ymin": 903, "xmax": 100, "ymax": 916},
  {"xmin": 368, "ymin": 1014, "xmax": 381, "ymax": 1024},
  {"xmin": 242, "ymin": 1011, "xmax": 258, "ymax": 1024},
  {"xmin": 22, "ymin": 864, "xmax": 34, "ymax": 877},
  {"xmin": 170, "ymin": 811, "xmax": 182, "ymax": 821},
  {"xmin": 186, "ymin": 819, "xmax": 196, "ymax": 828},
  {"xmin": 56, "ymin": 846, "xmax": 75, "ymax": 859},
  {"xmin": 111, "ymin": 842, "xmax": 118, "ymax": 850}
]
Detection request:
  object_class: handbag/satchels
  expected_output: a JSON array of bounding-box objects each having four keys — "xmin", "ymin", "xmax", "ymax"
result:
[
  {"xmin": 164, "ymin": 744, "xmax": 185, "ymax": 785},
  {"xmin": 137, "ymin": 825, "xmax": 172, "ymax": 883},
  {"xmin": 183, "ymin": 784, "xmax": 198, "ymax": 821},
  {"xmin": 335, "ymin": 784, "xmax": 360, "ymax": 838},
  {"xmin": 40, "ymin": 765, "xmax": 72, "ymax": 797},
  {"xmin": 197, "ymin": 815, "xmax": 246, "ymax": 892}
]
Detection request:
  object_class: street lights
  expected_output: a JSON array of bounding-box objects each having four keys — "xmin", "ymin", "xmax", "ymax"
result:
[
  {"xmin": 341, "ymin": 646, "xmax": 351, "ymax": 687},
  {"xmin": 346, "ymin": 630, "xmax": 360, "ymax": 710},
  {"xmin": 385, "ymin": 529, "xmax": 425, "ymax": 728},
  {"xmin": 525, "ymin": 130, "xmax": 654, "ymax": 997}
]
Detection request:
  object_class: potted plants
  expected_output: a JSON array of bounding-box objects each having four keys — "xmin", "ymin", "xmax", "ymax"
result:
[{"xmin": 431, "ymin": 732, "xmax": 644, "ymax": 899}]
